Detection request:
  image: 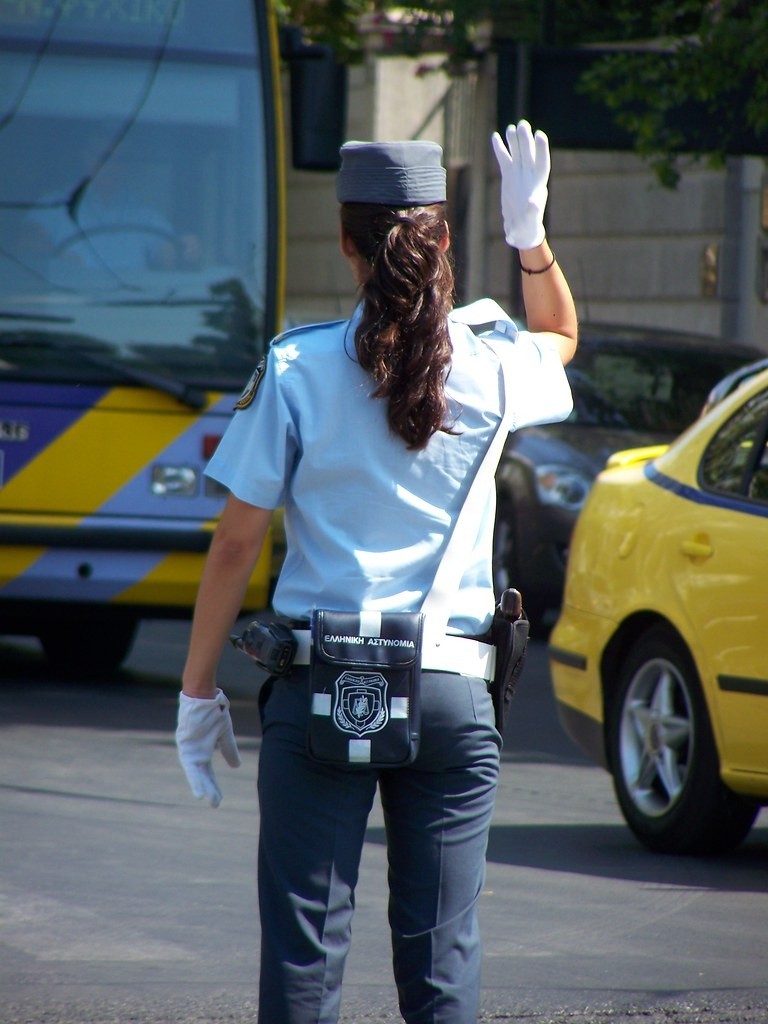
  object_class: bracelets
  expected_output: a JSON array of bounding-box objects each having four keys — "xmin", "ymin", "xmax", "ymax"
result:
[{"xmin": 520, "ymin": 251, "xmax": 556, "ymax": 276}]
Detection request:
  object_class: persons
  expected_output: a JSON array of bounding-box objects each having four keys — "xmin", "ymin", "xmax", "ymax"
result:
[{"xmin": 172, "ymin": 116, "xmax": 580, "ymax": 1018}]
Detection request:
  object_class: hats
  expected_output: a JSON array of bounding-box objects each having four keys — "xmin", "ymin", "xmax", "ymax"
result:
[{"xmin": 334, "ymin": 140, "xmax": 447, "ymax": 206}]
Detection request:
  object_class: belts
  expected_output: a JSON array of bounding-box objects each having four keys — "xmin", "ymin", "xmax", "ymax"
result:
[{"xmin": 292, "ymin": 630, "xmax": 497, "ymax": 683}]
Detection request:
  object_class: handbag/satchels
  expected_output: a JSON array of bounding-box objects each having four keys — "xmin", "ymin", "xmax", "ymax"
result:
[{"xmin": 307, "ymin": 609, "xmax": 426, "ymax": 770}]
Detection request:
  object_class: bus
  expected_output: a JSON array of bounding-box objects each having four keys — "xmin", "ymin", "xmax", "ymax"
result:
[{"xmin": 0, "ymin": 1, "xmax": 315, "ymax": 677}]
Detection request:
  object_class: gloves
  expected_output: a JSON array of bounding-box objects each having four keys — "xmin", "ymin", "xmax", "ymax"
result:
[
  {"xmin": 175, "ymin": 687, "xmax": 242, "ymax": 809},
  {"xmin": 491, "ymin": 121, "xmax": 550, "ymax": 251}
]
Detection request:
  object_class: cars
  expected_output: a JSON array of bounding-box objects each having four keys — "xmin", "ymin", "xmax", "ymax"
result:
[
  {"xmin": 545, "ymin": 358, "xmax": 768, "ymax": 858},
  {"xmin": 497, "ymin": 362, "xmax": 698, "ymax": 637}
]
